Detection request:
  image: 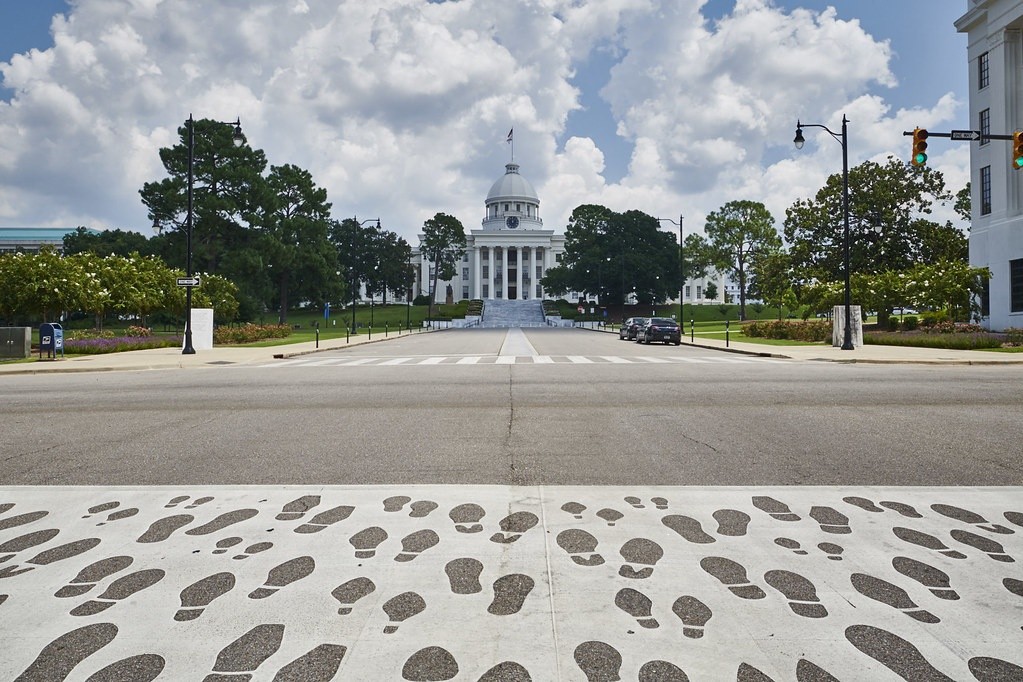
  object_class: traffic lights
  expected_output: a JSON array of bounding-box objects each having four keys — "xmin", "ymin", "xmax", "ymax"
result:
[
  {"xmin": 1013, "ymin": 131, "xmax": 1023, "ymax": 170},
  {"xmin": 912, "ymin": 129, "xmax": 929, "ymax": 166}
]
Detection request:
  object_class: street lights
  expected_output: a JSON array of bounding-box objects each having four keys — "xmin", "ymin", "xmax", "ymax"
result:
[
  {"xmin": 655, "ymin": 217, "xmax": 700, "ymax": 335},
  {"xmin": 396, "ymin": 252, "xmax": 427, "ymax": 328},
  {"xmin": 793, "ymin": 122, "xmax": 882, "ymax": 350},
  {"xmin": 586, "ymin": 268, "xmax": 610, "ymax": 327},
  {"xmin": 336, "ymin": 218, "xmax": 382, "ymax": 334},
  {"xmin": 607, "ymin": 256, "xmax": 636, "ymax": 324},
  {"xmin": 151, "ymin": 119, "xmax": 246, "ymax": 355},
  {"xmin": 419, "ymin": 262, "xmax": 451, "ymax": 326}
]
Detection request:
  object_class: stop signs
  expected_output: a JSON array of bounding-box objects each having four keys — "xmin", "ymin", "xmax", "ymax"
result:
[{"xmin": 578, "ymin": 306, "xmax": 583, "ymax": 312}]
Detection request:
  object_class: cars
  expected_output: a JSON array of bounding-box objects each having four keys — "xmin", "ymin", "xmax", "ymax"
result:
[
  {"xmin": 818, "ymin": 310, "xmax": 832, "ymax": 317},
  {"xmin": 636, "ymin": 317, "xmax": 681, "ymax": 345},
  {"xmin": 892, "ymin": 307, "xmax": 917, "ymax": 314},
  {"xmin": 620, "ymin": 318, "xmax": 647, "ymax": 340}
]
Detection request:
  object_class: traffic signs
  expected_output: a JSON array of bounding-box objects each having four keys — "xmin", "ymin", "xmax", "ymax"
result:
[
  {"xmin": 176, "ymin": 278, "xmax": 200, "ymax": 286},
  {"xmin": 950, "ymin": 129, "xmax": 980, "ymax": 141}
]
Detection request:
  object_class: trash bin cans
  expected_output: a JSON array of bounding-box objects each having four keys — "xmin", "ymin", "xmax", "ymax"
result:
[{"xmin": 40, "ymin": 323, "xmax": 63, "ymax": 350}]
